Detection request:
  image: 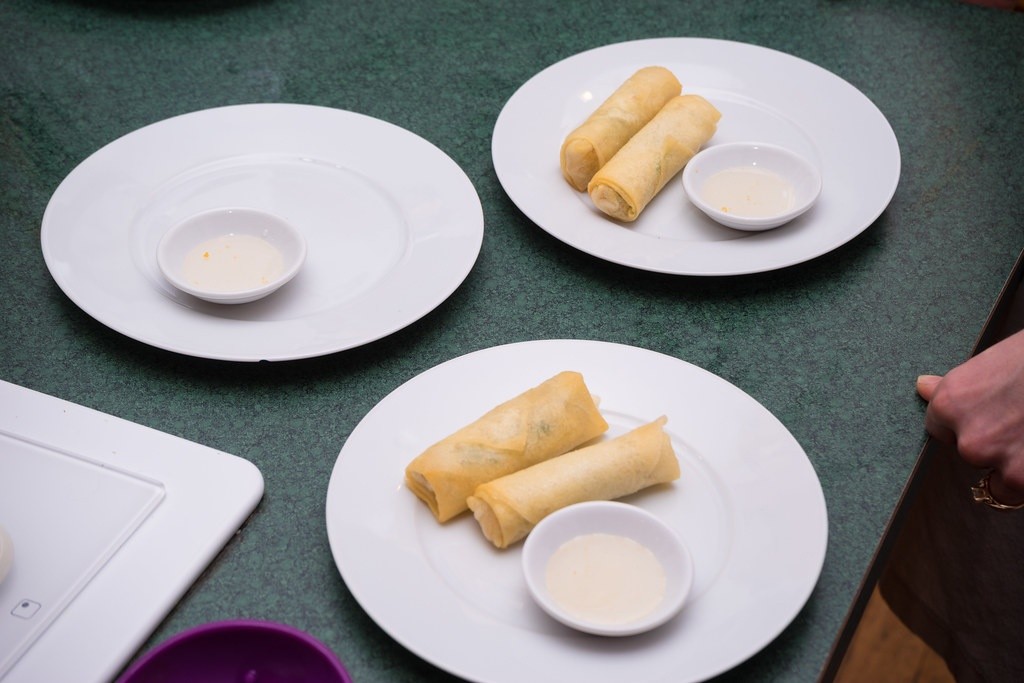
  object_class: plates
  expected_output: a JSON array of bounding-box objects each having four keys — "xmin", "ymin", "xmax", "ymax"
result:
[
  {"xmin": 521, "ymin": 501, "xmax": 692, "ymax": 636},
  {"xmin": 327, "ymin": 340, "xmax": 829, "ymax": 683},
  {"xmin": 683, "ymin": 142, "xmax": 823, "ymax": 231},
  {"xmin": 491, "ymin": 36, "xmax": 902, "ymax": 276},
  {"xmin": 159, "ymin": 209, "xmax": 305, "ymax": 304},
  {"xmin": 40, "ymin": 104, "xmax": 485, "ymax": 363}
]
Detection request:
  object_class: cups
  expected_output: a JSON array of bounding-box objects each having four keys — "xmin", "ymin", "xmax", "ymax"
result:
[{"xmin": 119, "ymin": 621, "xmax": 349, "ymax": 683}]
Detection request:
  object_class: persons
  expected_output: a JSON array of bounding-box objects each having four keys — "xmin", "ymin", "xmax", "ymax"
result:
[{"xmin": 875, "ymin": 327, "xmax": 1024, "ymax": 682}]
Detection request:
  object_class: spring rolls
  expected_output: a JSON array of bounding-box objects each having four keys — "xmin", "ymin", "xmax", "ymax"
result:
[
  {"xmin": 588, "ymin": 94, "xmax": 721, "ymax": 222},
  {"xmin": 466, "ymin": 415, "xmax": 681, "ymax": 549},
  {"xmin": 404, "ymin": 370, "xmax": 609, "ymax": 524},
  {"xmin": 562, "ymin": 65, "xmax": 682, "ymax": 193}
]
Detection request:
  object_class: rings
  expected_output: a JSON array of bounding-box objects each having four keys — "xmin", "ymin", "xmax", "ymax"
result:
[{"xmin": 970, "ymin": 478, "xmax": 1024, "ymax": 510}]
